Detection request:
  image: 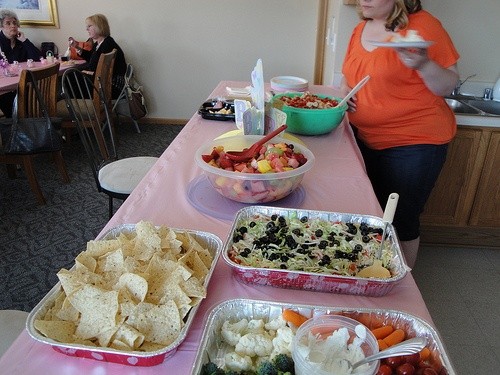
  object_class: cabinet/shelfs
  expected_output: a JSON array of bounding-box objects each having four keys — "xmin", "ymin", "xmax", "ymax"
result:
[{"xmin": 419, "ymin": 125, "xmax": 500, "ymax": 250}]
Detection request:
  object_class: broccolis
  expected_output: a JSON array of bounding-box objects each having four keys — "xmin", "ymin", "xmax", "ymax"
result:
[{"xmin": 203, "ymin": 317, "xmax": 298, "ymax": 375}]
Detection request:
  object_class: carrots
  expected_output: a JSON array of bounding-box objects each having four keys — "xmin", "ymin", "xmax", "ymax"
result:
[{"xmin": 283, "ymin": 310, "xmax": 430, "ymax": 360}]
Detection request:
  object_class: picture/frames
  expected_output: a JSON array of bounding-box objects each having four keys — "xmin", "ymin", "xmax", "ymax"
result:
[{"xmin": 0, "ymin": 0, "xmax": 60, "ymax": 30}]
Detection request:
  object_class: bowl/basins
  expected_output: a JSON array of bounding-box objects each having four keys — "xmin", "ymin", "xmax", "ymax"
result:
[
  {"xmin": 194, "ymin": 134, "xmax": 315, "ymax": 204},
  {"xmin": 188, "ymin": 298, "xmax": 457, "ymax": 375},
  {"xmin": 222, "ymin": 205, "xmax": 408, "ymax": 298},
  {"xmin": 269, "ymin": 92, "xmax": 349, "ymax": 136},
  {"xmin": 291, "ymin": 314, "xmax": 380, "ymax": 375},
  {"xmin": 25, "ymin": 222, "xmax": 223, "ymax": 367}
]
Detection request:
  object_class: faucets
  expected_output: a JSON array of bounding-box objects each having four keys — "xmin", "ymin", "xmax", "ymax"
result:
[{"xmin": 450, "ymin": 73, "xmax": 477, "ymax": 95}]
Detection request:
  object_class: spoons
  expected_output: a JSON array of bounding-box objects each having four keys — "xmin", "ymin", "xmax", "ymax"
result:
[{"xmin": 341, "ymin": 337, "xmax": 428, "ymax": 375}]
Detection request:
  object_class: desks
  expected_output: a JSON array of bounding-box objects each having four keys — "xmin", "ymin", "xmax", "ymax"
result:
[{"xmin": 0, "ymin": 58, "xmax": 461, "ymax": 375}]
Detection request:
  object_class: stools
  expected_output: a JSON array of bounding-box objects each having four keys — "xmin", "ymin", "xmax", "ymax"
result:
[{"xmin": 0, "ymin": 309, "xmax": 30, "ymax": 360}]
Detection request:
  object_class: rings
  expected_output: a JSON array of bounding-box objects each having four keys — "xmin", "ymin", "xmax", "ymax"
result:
[{"xmin": 405, "ymin": 58, "xmax": 411, "ymax": 62}]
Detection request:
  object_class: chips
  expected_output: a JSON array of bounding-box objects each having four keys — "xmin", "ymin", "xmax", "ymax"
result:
[{"xmin": 34, "ymin": 221, "xmax": 212, "ymax": 352}]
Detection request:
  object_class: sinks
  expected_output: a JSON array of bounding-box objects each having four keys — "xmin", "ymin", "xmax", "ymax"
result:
[
  {"xmin": 467, "ymin": 98, "xmax": 500, "ymax": 116},
  {"xmin": 442, "ymin": 98, "xmax": 480, "ymax": 115}
]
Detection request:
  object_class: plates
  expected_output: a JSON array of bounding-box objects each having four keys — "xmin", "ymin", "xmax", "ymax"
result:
[
  {"xmin": 368, "ymin": 40, "xmax": 432, "ymax": 48},
  {"xmin": 197, "ymin": 97, "xmax": 237, "ymax": 119},
  {"xmin": 270, "ymin": 75, "xmax": 309, "ymax": 96}
]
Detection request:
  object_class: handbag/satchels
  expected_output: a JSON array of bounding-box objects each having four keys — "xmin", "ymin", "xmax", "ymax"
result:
[
  {"xmin": 41, "ymin": 42, "xmax": 58, "ymax": 59},
  {"xmin": 69, "ymin": 37, "xmax": 93, "ymax": 60},
  {"xmin": 5, "ymin": 70, "xmax": 63, "ymax": 155},
  {"xmin": 124, "ymin": 63, "xmax": 148, "ymax": 121}
]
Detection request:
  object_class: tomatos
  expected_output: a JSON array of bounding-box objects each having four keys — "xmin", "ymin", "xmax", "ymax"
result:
[
  {"xmin": 280, "ymin": 95, "xmax": 338, "ymax": 109},
  {"xmin": 376, "ymin": 352, "xmax": 438, "ymax": 375}
]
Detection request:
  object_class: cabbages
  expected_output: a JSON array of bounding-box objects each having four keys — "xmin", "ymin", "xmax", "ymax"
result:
[{"xmin": 228, "ymin": 212, "xmax": 393, "ymax": 275}]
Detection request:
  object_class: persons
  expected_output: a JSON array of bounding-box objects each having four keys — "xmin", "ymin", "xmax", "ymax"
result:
[
  {"xmin": 60, "ymin": 14, "xmax": 126, "ymax": 99},
  {"xmin": 0, "ymin": 10, "xmax": 42, "ymax": 118},
  {"xmin": 338, "ymin": 0, "xmax": 460, "ymax": 272}
]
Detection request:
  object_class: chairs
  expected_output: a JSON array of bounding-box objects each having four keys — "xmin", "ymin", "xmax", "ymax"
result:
[
  {"xmin": 101, "ymin": 63, "xmax": 144, "ymax": 133},
  {"xmin": 60, "ymin": 67, "xmax": 159, "ymax": 221},
  {"xmin": 56, "ymin": 48, "xmax": 116, "ymax": 162},
  {"xmin": 0, "ymin": 62, "xmax": 72, "ymax": 205}
]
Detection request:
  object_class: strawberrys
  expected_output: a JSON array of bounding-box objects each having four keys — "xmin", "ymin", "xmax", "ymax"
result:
[{"xmin": 202, "ymin": 144, "xmax": 305, "ymax": 203}]
